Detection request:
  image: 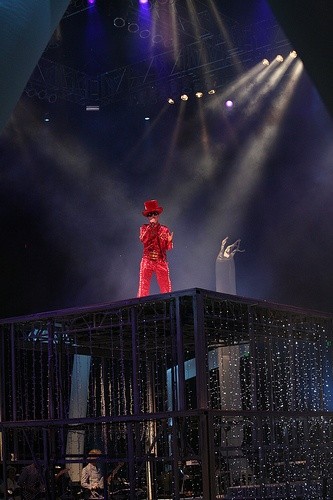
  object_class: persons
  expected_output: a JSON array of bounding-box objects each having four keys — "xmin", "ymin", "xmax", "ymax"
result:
[
  {"xmin": 81, "ymin": 449, "xmax": 108, "ymax": 500},
  {"xmin": 216, "ymin": 236, "xmax": 246, "ymax": 295},
  {"xmin": 17, "ymin": 463, "xmax": 68, "ymax": 500},
  {"xmin": 2, "ymin": 466, "xmax": 19, "ymax": 500},
  {"xmin": 135, "ymin": 199, "xmax": 174, "ymax": 298}
]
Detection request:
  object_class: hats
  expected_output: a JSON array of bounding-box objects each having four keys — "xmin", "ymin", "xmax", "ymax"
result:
[{"xmin": 143, "ymin": 199, "xmax": 163, "ymax": 217}]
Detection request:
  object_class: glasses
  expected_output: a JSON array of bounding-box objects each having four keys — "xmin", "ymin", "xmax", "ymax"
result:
[{"xmin": 147, "ymin": 211, "xmax": 159, "ymax": 218}]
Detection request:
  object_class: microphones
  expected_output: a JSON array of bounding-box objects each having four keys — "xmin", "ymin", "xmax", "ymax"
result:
[{"xmin": 151, "ymin": 222, "xmax": 155, "ymax": 227}]
